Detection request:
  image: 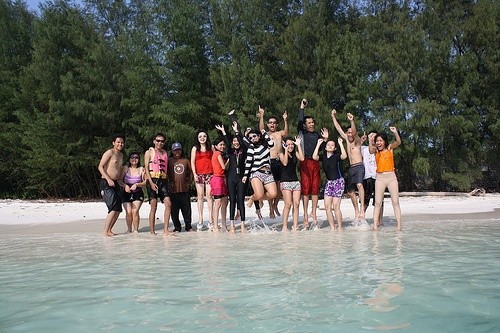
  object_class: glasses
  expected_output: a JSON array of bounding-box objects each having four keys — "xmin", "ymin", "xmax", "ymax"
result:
[
  {"xmin": 218, "ymin": 144, "xmax": 225, "ymax": 146},
  {"xmin": 268, "ymin": 121, "xmax": 277, "ymax": 124},
  {"xmin": 249, "ymin": 135, "xmax": 257, "ymax": 139},
  {"xmin": 155, "ymin": 139, "xmax": 164, "ymax": 143},
  {"xmin": 199, "ymin": 135, "xmax": 207, "ymax": 138}
]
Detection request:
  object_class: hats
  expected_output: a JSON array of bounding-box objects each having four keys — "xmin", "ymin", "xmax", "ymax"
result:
[{"xmin": 172, "ymin": 143, "xmax": 182, "ymax": 151}]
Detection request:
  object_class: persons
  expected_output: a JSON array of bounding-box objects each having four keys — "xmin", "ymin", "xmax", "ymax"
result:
[
  {"xmin": 98, "ymin": 136, "xmax": 124, "ymax": 238},
  {"xmin": 166, "ymin": 142, "xmax": 195, "ymax": 234},
  {"xmin": 331, "ymin": 109, "xmax": 365, "ymax": 222},
  {"xmin": 215, "ymin": 121, "xmax": 247, "ymax": 233},
  {"xmin": 312, "ymin": 139, "xmax": 348, "ymax": 231},
  {"xmin": 242, "ymin": 129, "xmax": 277, "ymax": 209},
  {"xmin": 258, "ymin": 104, "xmax": 288, "ymax": 219},
  {"xmin": 119, "ymin": 152, "xmax": 147, "ymax": 235},
  {"xmin": 367, "ymin": 126, "xmax": 402, "ymax": 233},
  {"xmin": 190, "ymin": 130, "xmax": 220, "ymax": 231},
  {"xmin": 277, "ymin": 135, "xmax": 304, "ymax": 235},
  {"xmin": 359, "ymin": 129, "xmax": 384, "ymax": 226},
  {"xmin": 227, "ymin": 109, "xmax": 263, "ymax": 222},
  {"xmin": 209, "ymin": 136, "xmax": 230, "ymax": 233},
  {"xmin": 143, "ymin": 133, "xmax": 176, "ymax": 237},
  {"xmin": 297, "ymin": 98, "xmax": 329, "ymax": 227}
]
